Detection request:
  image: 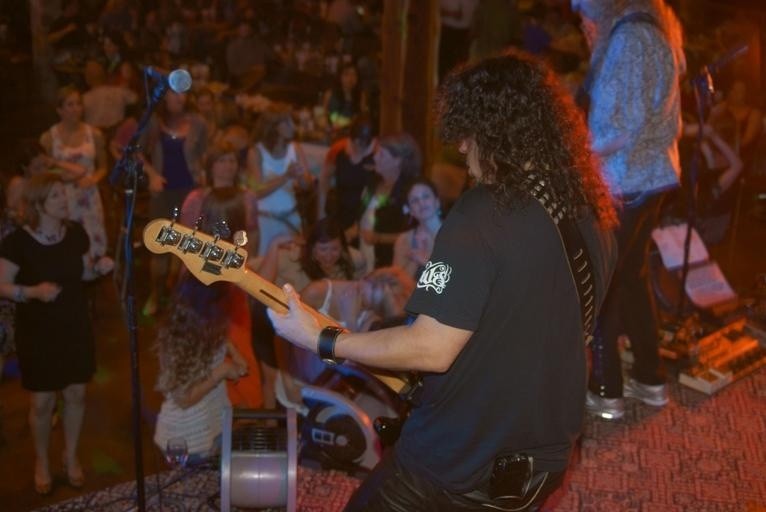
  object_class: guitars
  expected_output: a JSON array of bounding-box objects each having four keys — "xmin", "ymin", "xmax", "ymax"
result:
[{"xmin": 143, "ymin": 208, "xmax": 424, "ymax": 446}]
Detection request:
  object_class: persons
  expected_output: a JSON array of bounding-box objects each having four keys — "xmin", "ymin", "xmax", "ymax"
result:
[
  {"xmin": 268, "ymin": 54, "xmax": 618, "ymax": 512},
  {"xmin": 678, "ymin": 80, "xmax": 761, "ymax": 245},
  {"xmin": 573, "ymin": 0, "xmax": 686, "ymax": 420},
  {"xmin": 152, "ymin": 274, "xmax": 249, "ymax": 468},
  {"xmin": 0, "ymin": 0, "xmax": 443, "ymax": 429},
  {"xmin": 0, "ymin": 174, "xmax": 115, "ymax": 494}
]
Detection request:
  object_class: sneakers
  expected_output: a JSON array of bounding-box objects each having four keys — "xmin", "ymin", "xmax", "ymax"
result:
[
  {"xmin": 623, "ymin": 378, "xmax": 669, "ymax": 406},
  {"xmin": 585, "ymin": 391, "xmax": 625, "ymax": 419}
]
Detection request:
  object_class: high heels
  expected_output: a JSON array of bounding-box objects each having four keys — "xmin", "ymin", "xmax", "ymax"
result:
[{"xmin": 36, "ymin": 458, "xmax": 83, "ymax": 494}]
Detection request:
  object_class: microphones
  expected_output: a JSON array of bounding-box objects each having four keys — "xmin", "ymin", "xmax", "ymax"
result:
[
  {"xmin": 698, "ymin": 62, "xmax": 716, "ymax": 98},
  {"xmin": 143, "ymin": 64, "xmax": 192, "ymax": 94}
]
